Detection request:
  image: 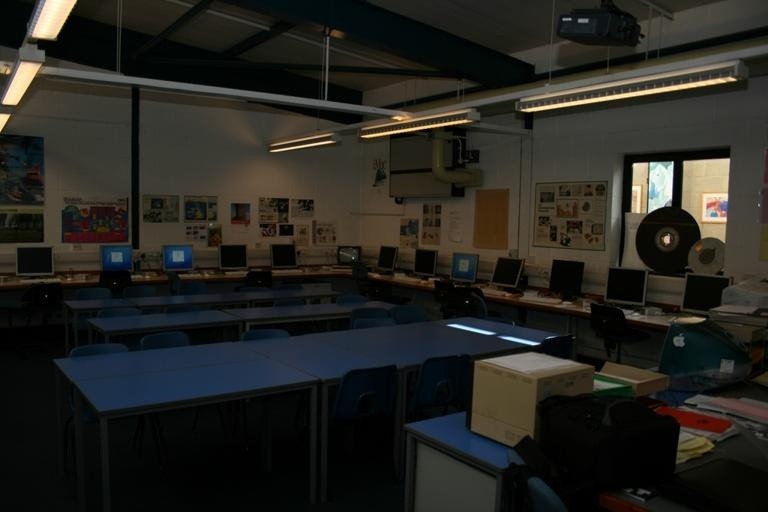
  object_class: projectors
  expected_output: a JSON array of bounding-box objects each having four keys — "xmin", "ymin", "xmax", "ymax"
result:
[{"xmin": 556, "ymin": 8, "xmax": 645, "ymax": 48}]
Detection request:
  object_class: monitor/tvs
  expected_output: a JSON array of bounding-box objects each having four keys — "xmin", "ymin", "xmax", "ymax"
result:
[
  {"xmin": 603, "ymin": 266, "xmax": 648, "ymax": 311},
  {"xmin": 376, "ymin": 246, "xmax": 397, "ymax": 274},
  {"xmin": 270, "ymin": 244, "xmax": 299, "ymax": 269},
  {"xmin": 16, "ymin": 245, "xmax": 54, "ymax": 276},
  {"xmin": 449, "ymin": 251, "xmax": 479, "ymax": 283},
  {"xmin": 414, "ymin": 248, "xmax": 437, "ymax": 276},
  {"xmin": 218, "ymin": 243, "xmax": 248, "ymax": 273},
  {"xmin": 161, "ymin": 244, "xmax": 194, "ymax": 272},
  {"xmin": 548, "ymin": 259, "xmax": 585, "ymax": 296},
  {"xmin": 337, "ymin": 246, "xmax": 361, "ymax": 266},
  {"xmin": 680, "ymin": 272, "xmax": 734, "ymax": 316},
  {"xmin": 489, "ymin": 256, "xmax": 525, "ymax": 288},
  {"xmin": 102, "ymin": 245, "xmax": 134, "ymax": 272}
]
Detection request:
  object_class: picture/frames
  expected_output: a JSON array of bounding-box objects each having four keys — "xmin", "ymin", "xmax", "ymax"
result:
[
  {"xmin": 631, "ymin": 184, "xmax": 643, "ymax": 213},
  {"xmin": 701, "ymin": 192, "xmax": 728, "ymax": 225}
]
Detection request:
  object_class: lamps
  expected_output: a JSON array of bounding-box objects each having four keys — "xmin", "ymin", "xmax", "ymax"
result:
[
  {"xmin": 358, "ymin": 64, "xmax": 481, "ymax": 141},
  {"xmin": 265, "ymin": 23, "xmax": 343, "ymax": 153},
  {"xmin": 514, "ymin": 0, "xmax": 747, "ymax": 114},
  {"xmin": 0, "ymin": 1, "xmax": 84, "ymax": 132}
]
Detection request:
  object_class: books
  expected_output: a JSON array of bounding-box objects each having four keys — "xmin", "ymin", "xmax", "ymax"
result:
[{"xmin": 594, "ymin": 364, "xmax": 670, "ymax": 396}]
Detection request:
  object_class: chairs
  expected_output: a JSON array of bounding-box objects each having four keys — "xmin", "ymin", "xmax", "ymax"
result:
[{"xmin": 0, "ymin": 261, "xmax": 707, "ymax": 511}]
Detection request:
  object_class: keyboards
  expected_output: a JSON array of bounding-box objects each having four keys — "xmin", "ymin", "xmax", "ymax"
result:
[
  {"xmin": 668, "ymin": 315, "xmax": 706, "ymax": 324},
  {"xmin": 485, "ymin": 289, "xmax": 507, "ymax": 296},
  {"xmin": 331, "ymin": 268, "xmax": 352, "ymax": 273},
  {"xmin": 21, "ymin": 278, "xmax": 64, "ymax": 284},
  {"xmin": 177, "ymin": 273, "xmax": 210, "ymax": 279},
  {"xmin": 131, "ymin": 274, "xmax": 158, "ymax": 279},
  {"xmin": 519, "ymin": 295, "xmax": 563, "ymax": 304},
  {"xmin": 616, "ymin": 306, "xmax": 635, "ymax": 315},
  {"xmin": 224, "ymin": 270, "xmax": 249, "ymax": 276},
  {"xmin": 272, "ymin": 269, "xmax": 303, "ymax": 273}
]
